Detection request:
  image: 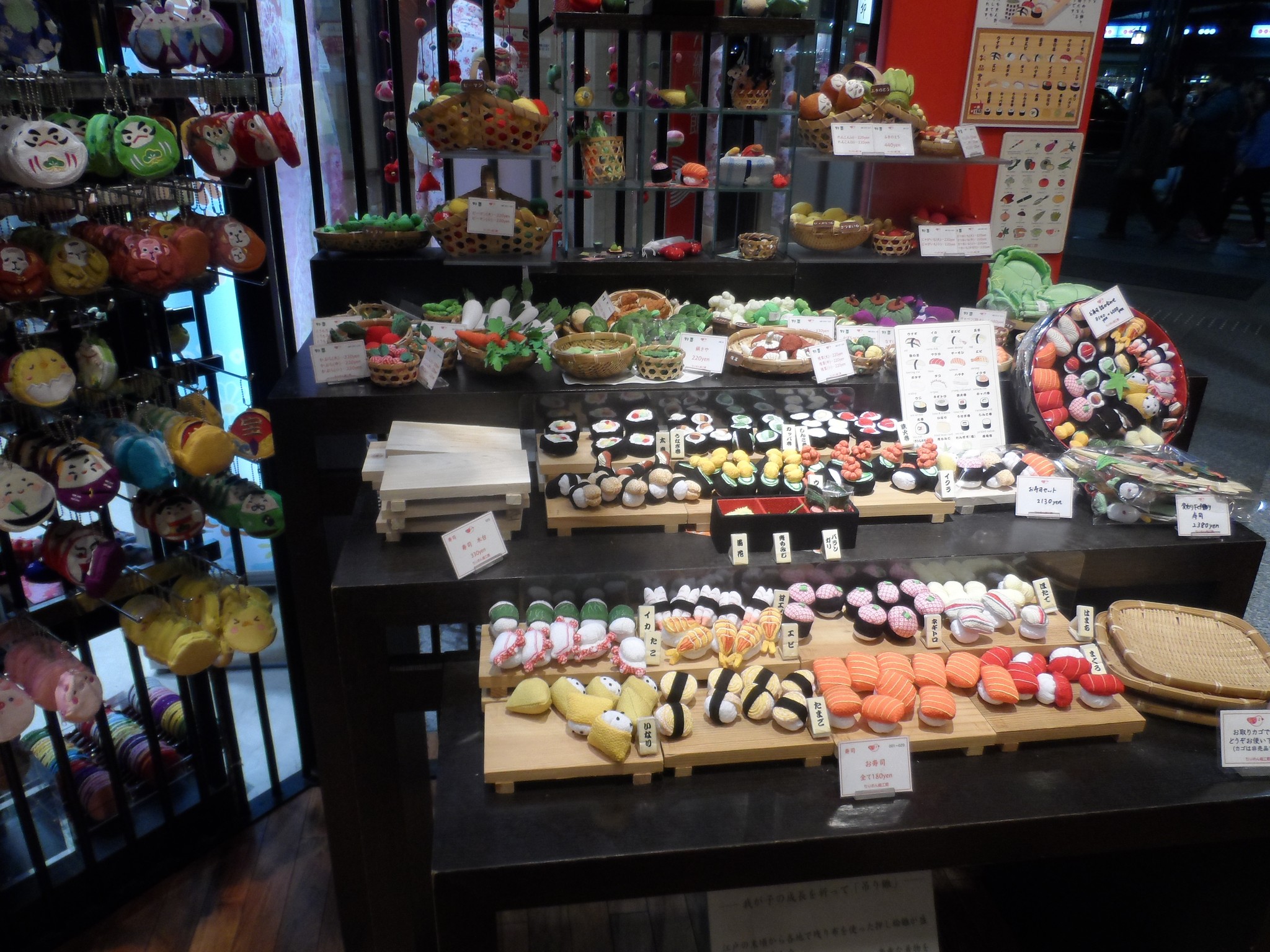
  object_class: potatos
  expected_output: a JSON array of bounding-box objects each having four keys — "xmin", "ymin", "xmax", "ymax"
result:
[{"xmin": 608, "ymin": 292, "xmax": 670, "ymax": 328}]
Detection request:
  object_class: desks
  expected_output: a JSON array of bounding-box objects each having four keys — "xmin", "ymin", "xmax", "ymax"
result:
[{"xmin": 268, "ymin": 294, "xmax": 1270, "ymax": 952}]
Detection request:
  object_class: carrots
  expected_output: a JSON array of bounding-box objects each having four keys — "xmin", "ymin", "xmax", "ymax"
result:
[
  {"xmin": 455, "ymin": 318, "xmax": 553, "ymax": 374},
  {"xmin": 329, "ymin": 312, "xmax": 456, "ymax": 365}
]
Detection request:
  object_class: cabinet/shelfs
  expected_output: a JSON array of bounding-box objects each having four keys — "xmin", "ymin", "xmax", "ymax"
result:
[{"xmin": 280, "ymin": 0, "xmax": 1025, "ymax": 312}]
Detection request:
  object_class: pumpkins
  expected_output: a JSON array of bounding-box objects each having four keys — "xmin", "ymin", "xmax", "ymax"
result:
[{"xmin": 830, "ymin": 291, "xmax": 913, "ymax": 325}]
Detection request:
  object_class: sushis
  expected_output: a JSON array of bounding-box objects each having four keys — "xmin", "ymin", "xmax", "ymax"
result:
[
  {"xmin": 540, "ymin": 408, "xmax": 1056, "ymax": 510},
  {"xmin": 1034, "ymin": 302, "xmax": 1181, "ymax": 446},
  {"xmin": 488, "ymin": 574, "xmax": 1129, "ymax": 760},
  {"xmin": 983, "ymin": 51, "xmax": 1086, "ymax": 117},
  {"xmin": 681, "ymin": 163, "xmax": 707, "ymax": 185}
]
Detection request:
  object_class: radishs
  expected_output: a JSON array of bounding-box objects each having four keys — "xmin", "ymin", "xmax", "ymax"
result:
[{"xmin": 461, "ymin": 278, "xmax": 571, "ymax": 330}]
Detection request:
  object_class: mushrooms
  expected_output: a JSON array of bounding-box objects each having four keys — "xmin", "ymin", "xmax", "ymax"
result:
[{"xmin": 738, "ymin": 331, "xmax": 814, "ymax": 360}]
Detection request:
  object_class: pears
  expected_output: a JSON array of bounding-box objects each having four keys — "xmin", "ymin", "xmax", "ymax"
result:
[{"xmin": 789, "ymin": 202, "xmax": 865, "ymax": 228}]
